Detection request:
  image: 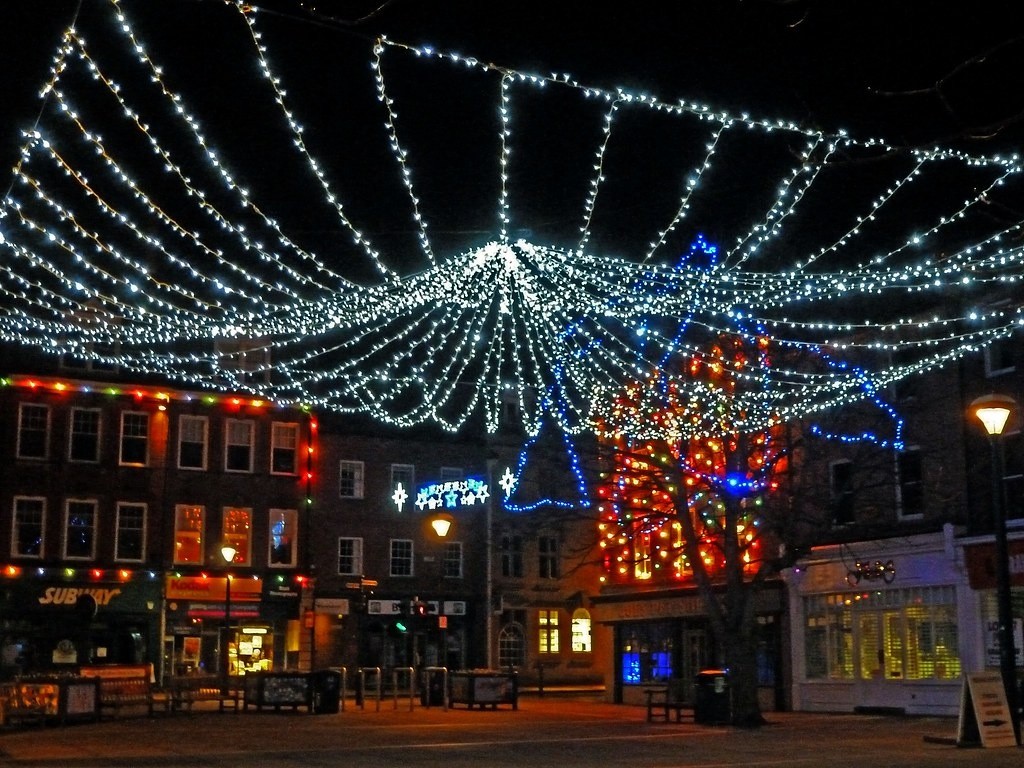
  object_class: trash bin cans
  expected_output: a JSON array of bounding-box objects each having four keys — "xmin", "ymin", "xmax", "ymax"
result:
[
  {"xmin": 312, "ymin": 669, "xmax": 342, "ymax": 714},
  {"xmin": 694, "ymin": 670, "xmax": 731, "ymax": 723},
  {"xmin": 420, "ymin": 667, "xmax": 447, "ymax": 706}
]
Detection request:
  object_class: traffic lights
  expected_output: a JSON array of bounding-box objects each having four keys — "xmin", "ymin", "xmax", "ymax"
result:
[
  {"xmin": 395, "ymin": 600, "xmax": 411, "ymax": 633},
  {"xmin": 415, "ymin": 600, "xmax": 426, "ymax": 617}
]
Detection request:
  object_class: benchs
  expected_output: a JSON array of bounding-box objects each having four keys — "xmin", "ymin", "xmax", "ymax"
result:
[
  {"xmin": 99, "ymin": 676, "xmax": 154, "ymax": 720},
  {"xmin": 170, "ymin": 675, "xmax": 238, "ymax": 716},
  {"xmin": 645, "ymin": 678, "xmax": 696, "ymax": 724}
]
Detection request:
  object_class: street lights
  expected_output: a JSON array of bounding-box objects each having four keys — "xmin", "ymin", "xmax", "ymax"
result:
[
  {"xmin": 965, "ymin": 391, "xmax": 1024, "ymax": 750},
  {"xmin": 427, "ymin": 512, "xmax": 456, "ymax": 685},
  {"xmin": 220, "ymin": 542, "xmax": 237, "ymax": 697}
]
